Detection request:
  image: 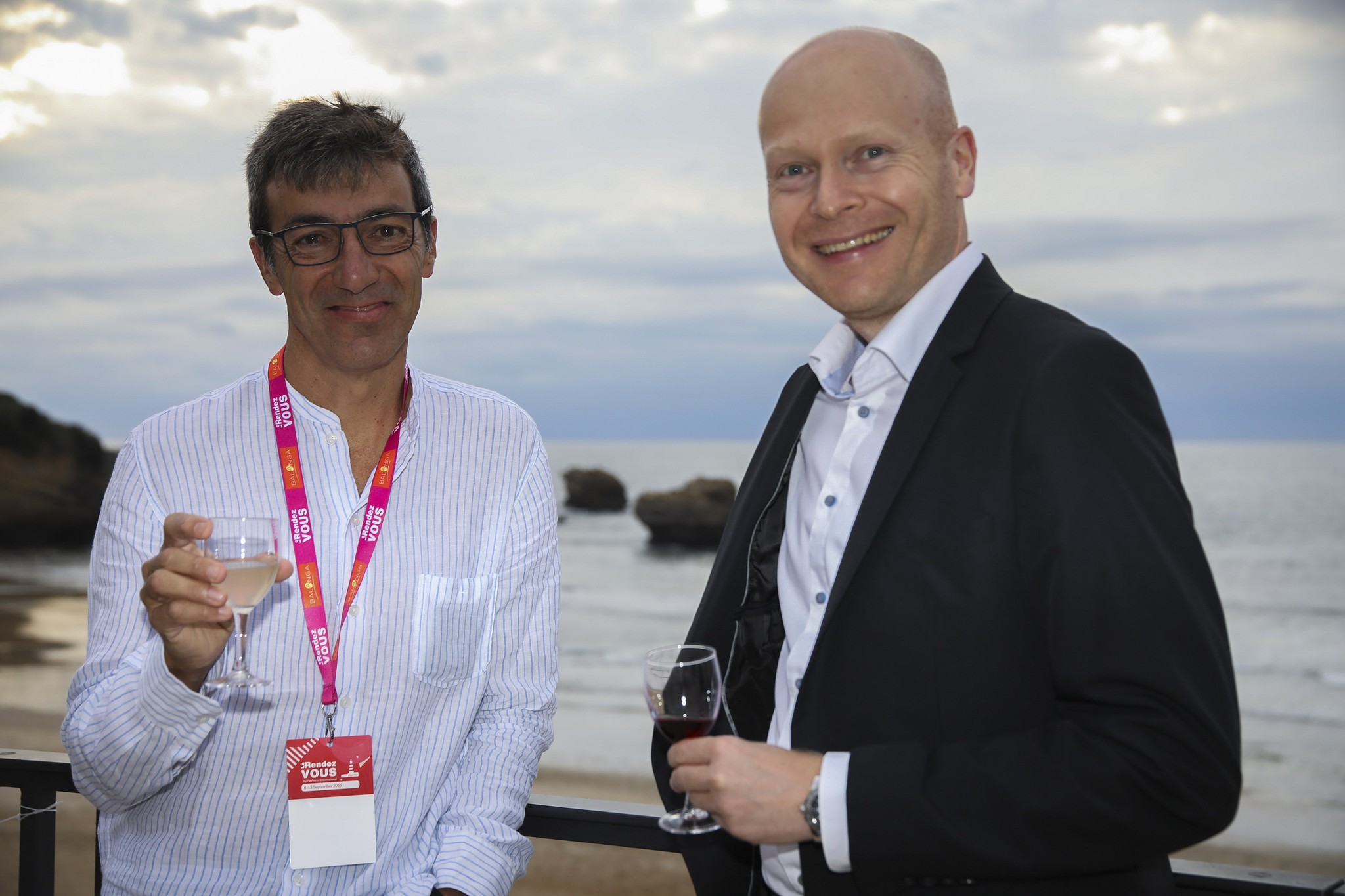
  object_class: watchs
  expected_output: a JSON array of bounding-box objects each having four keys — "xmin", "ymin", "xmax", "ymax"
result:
[{"xmin": 800, "ymin": 774, "xmax": 822, "ymax": 837}]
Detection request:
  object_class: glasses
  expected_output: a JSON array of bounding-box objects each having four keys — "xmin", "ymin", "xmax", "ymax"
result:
[{"xmin": 255, "ymin": 204, "xmax": 434, "ymax": 267}]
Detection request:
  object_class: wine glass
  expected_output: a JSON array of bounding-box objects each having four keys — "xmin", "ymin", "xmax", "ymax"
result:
[
  {"xmin": 640, "ymin": 643, "xmax": 737, "ymax": 837},
  {"xmin": 203, "ymin": 512, "xmax": 279, "ymax": 689}
]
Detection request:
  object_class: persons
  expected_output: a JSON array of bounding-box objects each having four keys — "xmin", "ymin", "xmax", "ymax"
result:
[
  {"xmin": 58, "ymin": 87, "xmax": 566, "ymax": 896},
  {"xmin": 650, "ymin": 25, "xmax": 1244, "ymax": 896}
]
